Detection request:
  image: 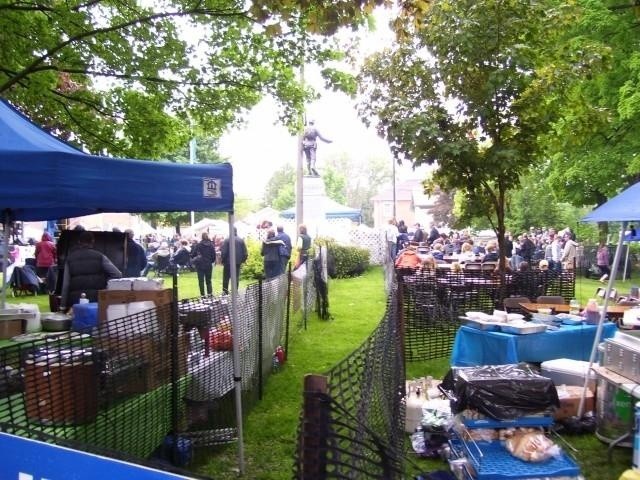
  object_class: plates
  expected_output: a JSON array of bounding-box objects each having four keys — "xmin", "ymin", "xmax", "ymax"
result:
[
  {"xmin": 556, "ymin": 312, "xmax": 585, "ymax": 325},
  {"xmin": 106, "ymin": 300, "xmax": 164, "ymax": 335}
]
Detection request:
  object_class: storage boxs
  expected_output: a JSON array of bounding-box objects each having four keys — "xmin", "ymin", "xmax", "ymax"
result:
[
  {"xmin": 541, "ymin": 358, "xmax": 600, "ymax": 419},
  {"xmin": 94, "ymin": 286, "xmax": 189, "ymax": 390},
  {"xmin": 0, "ymin": 320, "xmax": 25, "ymax": 340}
]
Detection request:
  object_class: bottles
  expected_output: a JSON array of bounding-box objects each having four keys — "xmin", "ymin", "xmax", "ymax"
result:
[
  {"xmin": 586, "ymin": 298, "xmax": 599, "ymax": 325},
  {"xmin": 271, "ymin": 344, "xmax": 284, "ymax": 373},
  {"xmin": 179, "ymin": 401, "xmax": 210, "ymax": 433},
  {"xmin": 569, "ymin": 300, "xmax": 580, "ymax": 316},
  {"xmin": 408, "ymin": 375, "xmax": 434, "ymax": 400}
]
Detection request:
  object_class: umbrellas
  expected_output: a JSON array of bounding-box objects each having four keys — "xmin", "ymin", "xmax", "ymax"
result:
[{"xmin": 580, "ymin": 181, "xmax": 640, "ymax": 222}]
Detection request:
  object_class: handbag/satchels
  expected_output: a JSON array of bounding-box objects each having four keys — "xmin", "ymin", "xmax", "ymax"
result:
[{"xmin": 190, "ymin": 252, "xmax": 202, "ymax": 264}]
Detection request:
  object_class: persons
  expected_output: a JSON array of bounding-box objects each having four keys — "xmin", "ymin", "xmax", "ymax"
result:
[
  {"xmin": 8, "ymin": 218, "xmax": 293, "ymax": 318},
  {"xmin": 596, "ymin": 239, "xmax": 610, "ymax": 283},
  {"xmin": 294, "ymin": 223, "xmax": 311, "ymax": 273},
  {"xmin": 303, "ymin": 121, "xmax": 332, "ymax": 176},
  {"xmin": 630, "ymin": 225, "xmax": 637, "ymax": 241},
  {"xmin": 388, "ymin": 216, "xmax": 579, "ymax": 296}
]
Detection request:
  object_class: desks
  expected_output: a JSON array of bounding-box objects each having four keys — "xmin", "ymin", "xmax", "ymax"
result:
[
  {"xmin": 460, "ymin": 321, "xmax": 616, "ymax": 363},
  {"xmin": 0, "ymin": 346, "xmax": 250, "ymax": 461}
]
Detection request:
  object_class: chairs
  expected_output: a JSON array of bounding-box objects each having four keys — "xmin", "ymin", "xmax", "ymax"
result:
[
  {"xmin": 154, "ymin": 249, "xmax": 192, "ymax": 277},
  {"xmin": 397, "ymin": 241, "xmax": 640, "ymax": 329}
]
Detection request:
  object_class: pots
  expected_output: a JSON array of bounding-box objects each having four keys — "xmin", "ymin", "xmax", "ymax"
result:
[{"xmin": 40, "ymin": 311, "xmax": 72, "ymax": 331}]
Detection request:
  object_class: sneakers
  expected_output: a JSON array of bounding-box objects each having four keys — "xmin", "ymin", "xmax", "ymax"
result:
[{"xmin": 202, "ymin": 294, "xmax": 213, "ymax": 300}]
